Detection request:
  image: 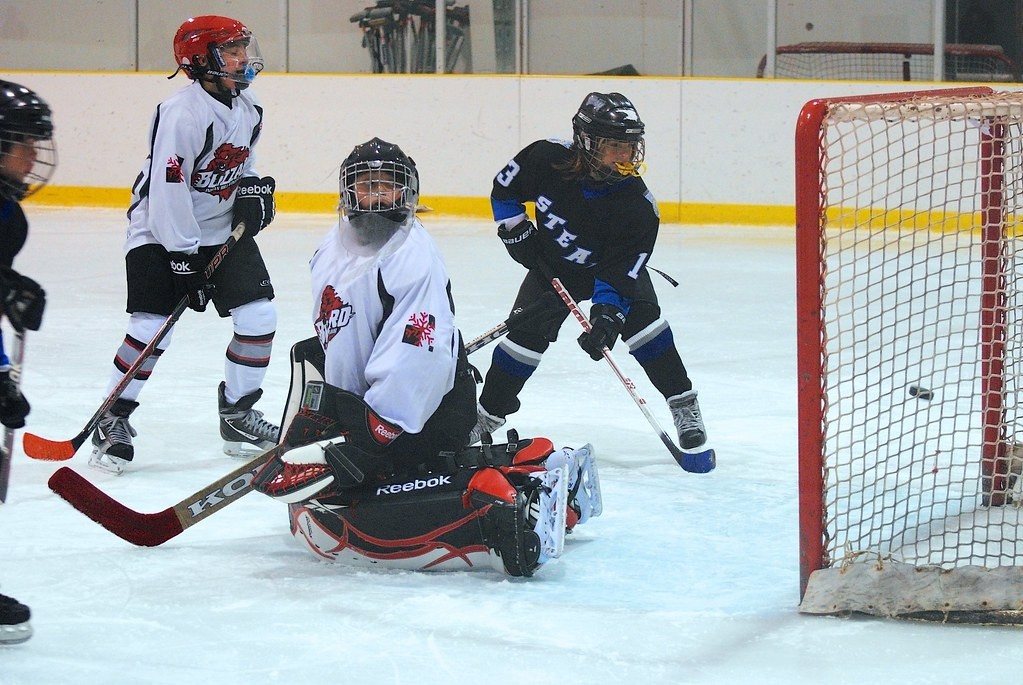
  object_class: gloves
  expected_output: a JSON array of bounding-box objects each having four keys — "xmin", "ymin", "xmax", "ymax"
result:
[
  {"xmin": 0, "ymin": 267, "xmax": 46, "ymax": 333},
  {"xmin": 577, "ymin": 304, "xmax": 627, "ymax": 361},
  {"xmin": 497, "ymin": 220, "xmax": 543, "ymax": 269},
  {"xmin": 231, "ymin": 176, "xmax": 276, "ymax": 237},
  {"xmin": 167, "ymin": 251, "xmax": 213, "ymax": 312},
  {"xmin": 1, "ymin": 363, "xmax": 29, "ymax": 429}
]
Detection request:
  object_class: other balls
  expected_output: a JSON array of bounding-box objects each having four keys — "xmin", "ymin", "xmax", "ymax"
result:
[{"xmin": 909, "ymin": 385, "xmax": 935, "ymax": 401}]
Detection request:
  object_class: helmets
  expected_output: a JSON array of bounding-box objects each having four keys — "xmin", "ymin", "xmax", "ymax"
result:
[
  {"xmin": 0, "ymin": 79, "xmax": 57, "ymax": 201},
  {"xmin": 337, "ymin": 137, "xmax": 420, "ymax": 232},
  {"xmin": 173, "ymin": 15, "xmax": 250, "ymax": 79},
  {"xmin": 573, "ymin": 92, "xmax": 645, "ymax": 189}
]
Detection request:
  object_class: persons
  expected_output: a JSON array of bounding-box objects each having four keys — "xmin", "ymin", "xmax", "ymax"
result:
[
  {"xmin": 88, "ymin": 16, "xmax": 280, "ymax": 474},
  {"xmin": 0, "ymin": 79, "xmax": 57, "ymax": 642},
  {"xmin": 461, "ymin": 93, "xmax": 708, "ymax": 450},
  {"xmin": 309, "ymin": 135, "xmax": 601, "ymax": 575}
]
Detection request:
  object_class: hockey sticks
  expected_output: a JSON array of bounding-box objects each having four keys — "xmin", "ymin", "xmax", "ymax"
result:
[
  {"xmin": 1, "ymin": 289, "xmax": 32, "ymax": 506},
  {"xmin": 535, "ymin": 256, "xmax": 720, "ymax": 475},
  {"xmin": 44, "ymin": 291, "xmax": 559, "ymax": 548},
  {"xmin": 22, "ymin": 221, "xmax": 248, "ymax": 462},
  {"xmin": 348, "ymin": 0, "xmax": 470, "ymax": 72}
]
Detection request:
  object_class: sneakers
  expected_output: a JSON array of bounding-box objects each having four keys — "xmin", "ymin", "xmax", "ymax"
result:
[
  {"xmin": 503, "ymin": 464, "xmax": 570, "ymax": 577},
  {"xmin": 86, "ymin": 398, "xmax": 139, "ymax": 476},
  {"xmin": 667, "ymin": 390, "xmax": 708, "ymax": 448},
  {"xmin": 464, "ymin": 401, "xmax": 506, "ymax": 445},
  {"xmin": 0, "ymin": 594, "xmax": 34, "ymax": 644},
  {"xmin": 217, "ymin": 380, "xmax": 280, "ymax": 459},
  {"xmin": 541, "ymin": 442, "xmax": 603, "ymax": 530}
]
